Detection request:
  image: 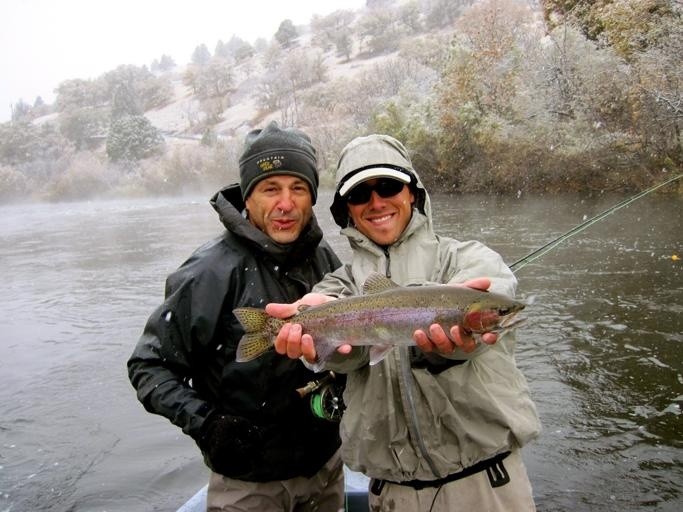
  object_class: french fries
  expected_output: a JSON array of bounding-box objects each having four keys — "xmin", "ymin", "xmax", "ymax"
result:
[{"xmin": 296, "ymin": 175, "xmax": 683, "ymax": 420}]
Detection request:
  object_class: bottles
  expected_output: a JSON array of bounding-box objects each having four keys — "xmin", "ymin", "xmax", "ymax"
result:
[
  {"xmin": 338, "ymin": 167, "xmax": 412, "ymax": 198},
  {"xmin": 238, "ymin": 120, "xmax": 319, "ymax": 207}
]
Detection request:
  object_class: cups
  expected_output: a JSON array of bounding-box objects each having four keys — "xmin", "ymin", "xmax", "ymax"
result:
[{"xmin": 342, "ymin": 178, "xmax": 406, "ymax": 206}]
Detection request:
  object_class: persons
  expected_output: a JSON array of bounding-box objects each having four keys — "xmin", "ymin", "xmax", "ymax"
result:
[
  {"xmin": 266, "ymin": 134, "xmax": 542, "ymax": 512},
  {"xmin": 127, "ymin": 121, "xmax": 347, "ymax": 512}
]
